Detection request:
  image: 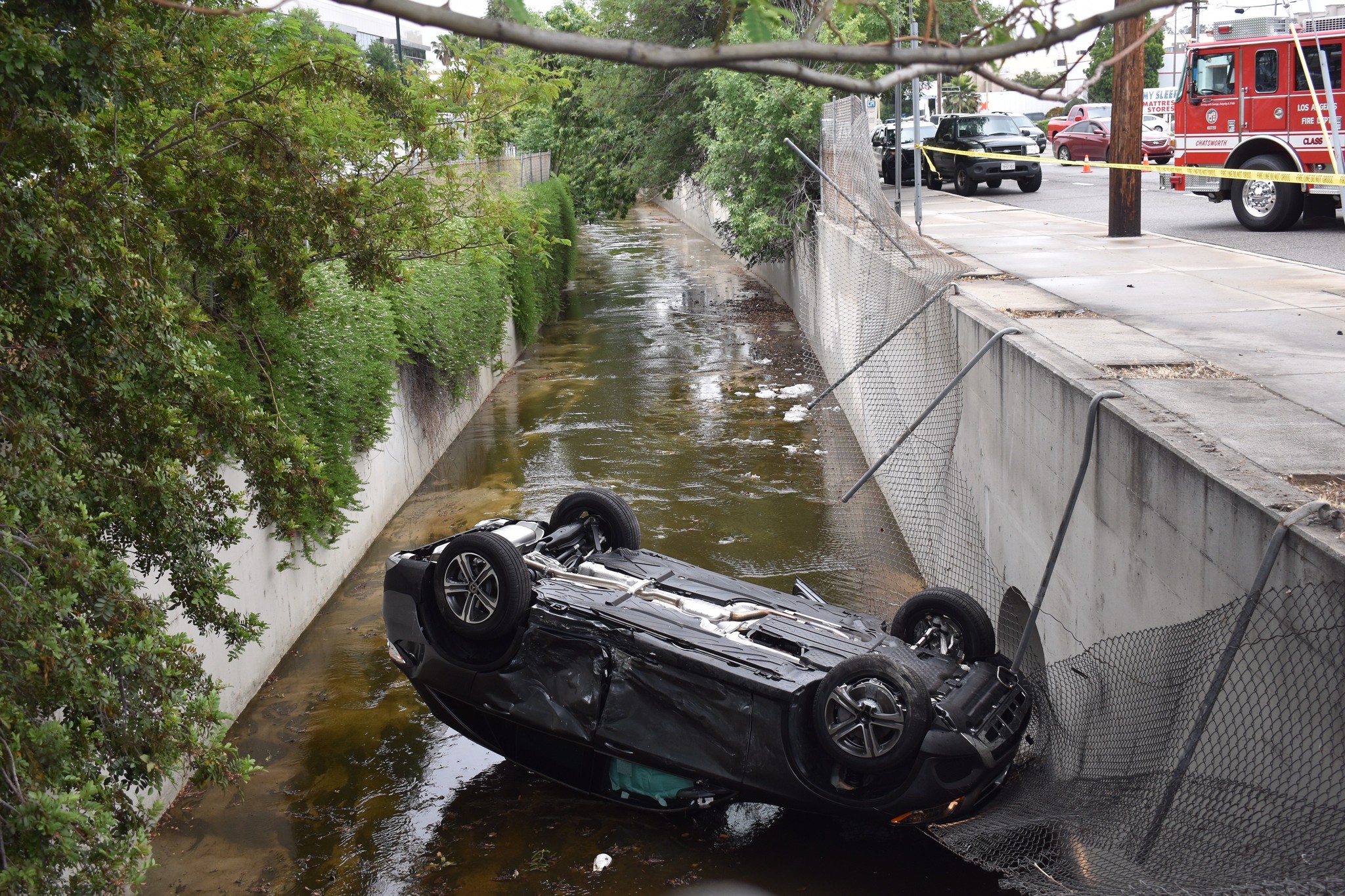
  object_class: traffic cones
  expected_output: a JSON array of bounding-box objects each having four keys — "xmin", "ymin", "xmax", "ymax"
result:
[
  {"xmin": 1141, "ymin": 153, "xmax": 1153, "ymax": 173},
  {"xmin": 1081, "ymin": 155, "xmax": 1093, "ymax": 173}
]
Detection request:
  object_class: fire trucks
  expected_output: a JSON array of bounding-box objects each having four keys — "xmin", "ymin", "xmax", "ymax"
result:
[{"xmin": 1159, "ymin": 14, "xmax": 1345, "ymax": 232}]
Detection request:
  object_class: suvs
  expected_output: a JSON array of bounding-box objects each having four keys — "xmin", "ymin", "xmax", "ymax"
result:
[
  {"xmin": 991, "ymin": 112, "xmax": 1047, "ymax": 154},
  {"xmin": 870, "ymin": 120, "xmax": 938, "ymax": 186}
]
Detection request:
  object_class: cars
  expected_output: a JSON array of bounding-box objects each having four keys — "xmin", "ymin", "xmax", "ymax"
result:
[
  {"xmin": 1142, "ymin": 114, "xmax": 1169, "ymax": 132},
  {"xmin": 884, "ymin": 114, "xmax": 972, "ymax": 127},
  {"xmin": 1052, "ymin": 116, "xmax": 1174, "ymax": 167},
  {"xmin": 381, "ymin": 486, "xmax": 1034, "ymax": 827}
]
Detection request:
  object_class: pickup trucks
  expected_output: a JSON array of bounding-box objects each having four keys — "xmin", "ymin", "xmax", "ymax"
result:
[
  {"xmin": 1047, "ymin": 103, "xmax": 1112, "ymax": 143},
  {"xmin": 921, "ymin": 111, "xmax": 1042, "ymax": 198}
]
